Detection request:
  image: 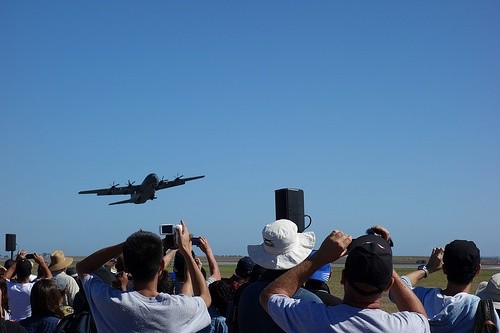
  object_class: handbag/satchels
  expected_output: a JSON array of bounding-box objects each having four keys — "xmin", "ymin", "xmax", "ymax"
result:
[{"xmin": 474, "ymin": 299, "xmax": 496, "ymax": 333}]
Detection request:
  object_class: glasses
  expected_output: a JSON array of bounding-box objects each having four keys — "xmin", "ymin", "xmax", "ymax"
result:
[{"xmin": 367, "ymin": 227, "xmax": 393, "ymax": 247}]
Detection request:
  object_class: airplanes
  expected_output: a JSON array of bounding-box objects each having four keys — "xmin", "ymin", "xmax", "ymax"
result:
[{"xmin": 79, "ymin": 174, "xmax": 207, "ymax": 205}]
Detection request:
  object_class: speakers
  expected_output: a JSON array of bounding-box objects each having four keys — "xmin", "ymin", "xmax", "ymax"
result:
[
  {"xmin": 275, "ymin": 189, "xmax": 304, "ymax": 233},
  {"xmin": 6, "ymin": 234, "xmax": 16, "ymax": 251}
]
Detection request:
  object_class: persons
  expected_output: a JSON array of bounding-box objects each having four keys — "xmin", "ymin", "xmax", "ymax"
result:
[
  {"xmin": 259, "ymin": 226, "xmax": 430, "ymax": 333},
  {"xmin": 76, "ymin": 219, "xmax": 211, "ymax": 333},
  {"xmin": 239, "ymin": 219, "xmax": 324, "ymax": 333},
  {"xmin": 389, "ymin": 240, "xmax": 500, "ymax": 333},
  {"xmin": 0, "ymin": 237, "xmax": 343, "ymax": 333}
]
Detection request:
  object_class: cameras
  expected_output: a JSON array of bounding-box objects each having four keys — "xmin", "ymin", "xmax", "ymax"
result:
[
  {"xmin": 159, "ymin": 223, "xmax": 183, "ymax": 244},
  {"xmin": 346, "ymin": 228, "xmax": 384, "ymax": 251},
  {"xmin": 25, "ymin": 254, "xmax": 36, "ymax": 259},
  {"xmin": 190, "ymin": 238, "xmax": 201, "ymax": 245}
]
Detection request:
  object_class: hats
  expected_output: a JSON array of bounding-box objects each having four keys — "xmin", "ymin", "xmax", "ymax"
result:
[
  {"xmin": 443, "ymin": 240, "xmax": 480, "ymax": 272},
  {"xmin": 237, "ymin": 257, "xmax": 257, "ymax": 273},
  {"xmin": 475, "ymin": 273, "xmax": 500, "ymax": 302},
  {"xmin": 345, "ymin": 235, "xmax": 392, "ymax": 296},
  {"xmin": 305, "ymin": 250, "xmax": 331, "ymax": 283},
  {"xmin": 48, "ymin": 250, "xmax": 73, "ymax": 271},
  {"xmin": 247, "ymin": 219, "xmax": 315, "ymax": 270}
]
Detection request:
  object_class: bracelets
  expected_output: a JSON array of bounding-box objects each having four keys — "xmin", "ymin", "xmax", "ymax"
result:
[{"xmin": 417, "ymin": 265, "xmax": 430, "ymax": 277}]
